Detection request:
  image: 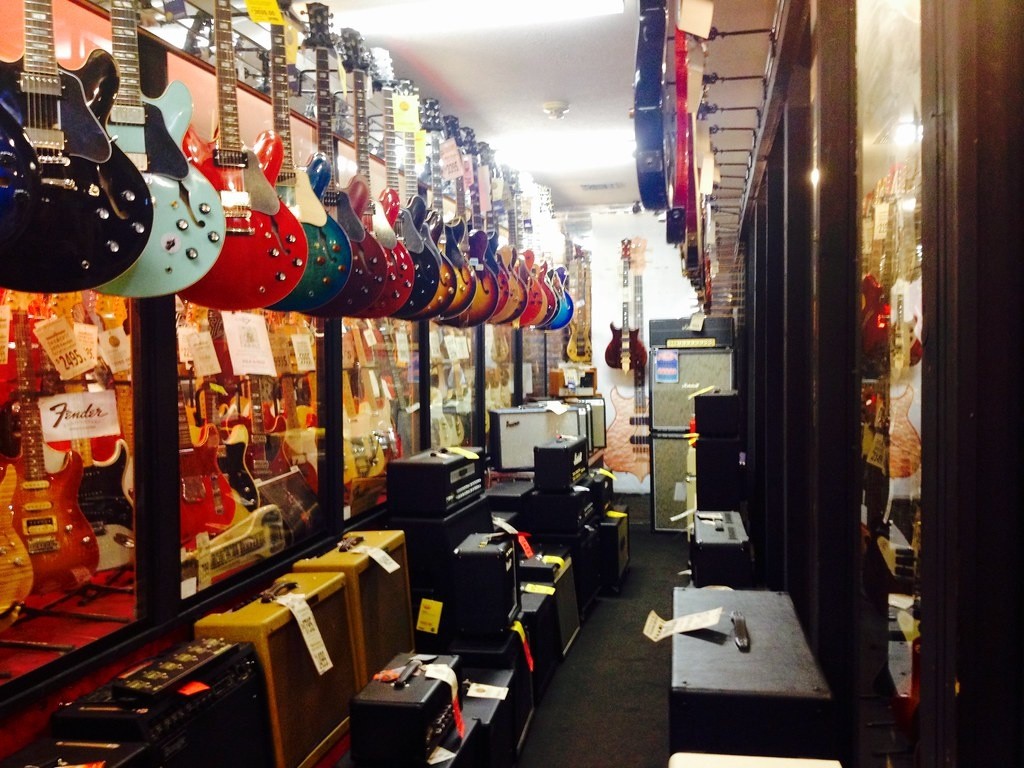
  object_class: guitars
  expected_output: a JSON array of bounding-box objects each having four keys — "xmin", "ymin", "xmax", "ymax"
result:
[
  {"xmin": 605, "ymin": 237, "xmax": 647, "ymax": 374},
  {"xmin": 0, "ymin": 1, "xmax": 589, "ymax": 616},
  {"xmin": 888, "ymin": 291, "xmax": 922, "ymax": 480},
  {"xmin": 603, "ymin": 234, "xmax": 651, "ymax": 485},
  {"xmin": 891, "ymin": 295, "xmax": 922, "ymax": 380}
]
